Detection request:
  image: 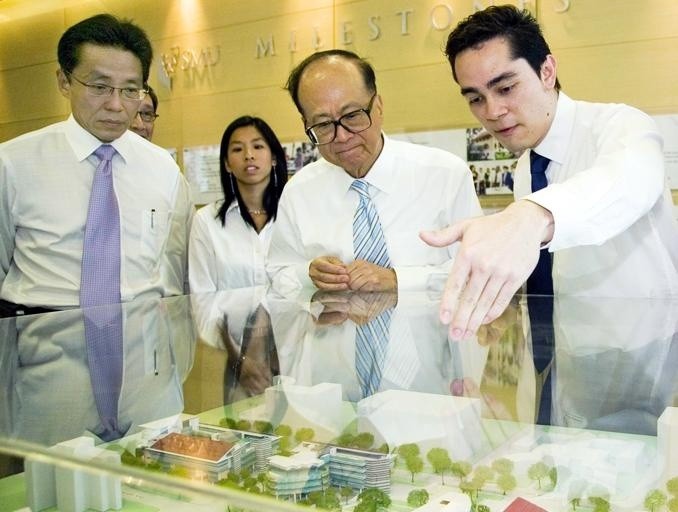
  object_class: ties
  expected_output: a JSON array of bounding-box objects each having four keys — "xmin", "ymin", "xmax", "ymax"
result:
[
  {"xmin": 79, "ymin": 308, "xmax": 126, "ymax": 444},
  {"xmin": 346, "ymin": 180, "xmax": 393, "ymax": 270},
  {"xmin": 525, "ymin": 296, "xmax": 554, "ymax": 426},
  {"xmin": 80, "ymin": 147, "xmax": 124, "ymax": 307},
  {"xmin": 527, "ymin": 150, "xmax": 553, "ymax": 294},
  {"xmin": 352, "ymin": 310, "xmax": 393, "ymax": 399}
]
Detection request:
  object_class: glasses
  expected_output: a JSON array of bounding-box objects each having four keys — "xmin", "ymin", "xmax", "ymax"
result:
[
  {"xmin": 305, "ymin": 90, "xmax": 377, "ymax": 147},
  {"xmin": 64, "ymin": 70, "xmax": 150, "ymax": 100},
  {"xmin": 136, "ymin": 111, "xmax": 163, "ymax": 123}
]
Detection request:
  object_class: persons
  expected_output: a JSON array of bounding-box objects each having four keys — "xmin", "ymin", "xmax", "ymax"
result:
[
  {"xmin": 261, "ymin": 48, "xmax": 497, "ymax": 420},
  {"xmin": 281, "ymin": 141, "xmax": 320, "ymax": 174},
  {"xmin": 416, "ymin": 4, "xmax": 678, "ymax": 440},
  {"xmin": 465, "ymin": 126, "xmax": 518, "ymax": 196},
  {"xmin": 126, "ymin": 80, "xmax": 197, "ymax": 386},
  {"xmin": 258, "ymin": 290, "xmax": 489, "ymax": 509},
  {"xmin": 187, "ymin": 114, "xmax": 296, "ymax": 413},
  {"xmin": 0, "ymin": 15, "xmax": 181, "ymax": 479},
  {"xmin": 456, "ymin": 292, "xmax": 678, "ymax": 512},
  {"xmin": 186, "ymin": 282, "xmax": 292, "ymax": 439}
]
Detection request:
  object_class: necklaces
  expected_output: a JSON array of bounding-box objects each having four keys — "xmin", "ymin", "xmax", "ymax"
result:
[{"xmin": 243, "ymin": 208, "xmax": 267, "ymax": 217}]
